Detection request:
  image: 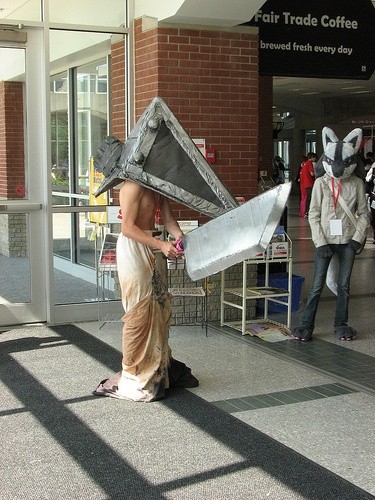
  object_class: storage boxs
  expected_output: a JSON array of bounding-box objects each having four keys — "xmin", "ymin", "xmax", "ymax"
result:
[{"xmin": 258, "ymin": 272, "xmax": 305, "ymax": 313}]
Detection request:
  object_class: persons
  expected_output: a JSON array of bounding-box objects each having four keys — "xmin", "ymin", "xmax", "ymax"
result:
[
  {"xmin": 91, "ymin": 181, "xmax": 189, "ymax": 403},
  {"xmin": 277, "ymin": 127, "xmax": 375, "ymax": 341}
]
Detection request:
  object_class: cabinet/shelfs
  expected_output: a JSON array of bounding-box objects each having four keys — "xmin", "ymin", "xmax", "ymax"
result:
[
  {"xmin": 94, "ymin": 232, "xmax": 124, "ymax": 330},
  {"xmin": 166, "ymin": 237, "xmax": 208, "ymax": 338},
  {"xmin": 220, "ymin": 230, "xmax": 293, "ymax": 335}
]
